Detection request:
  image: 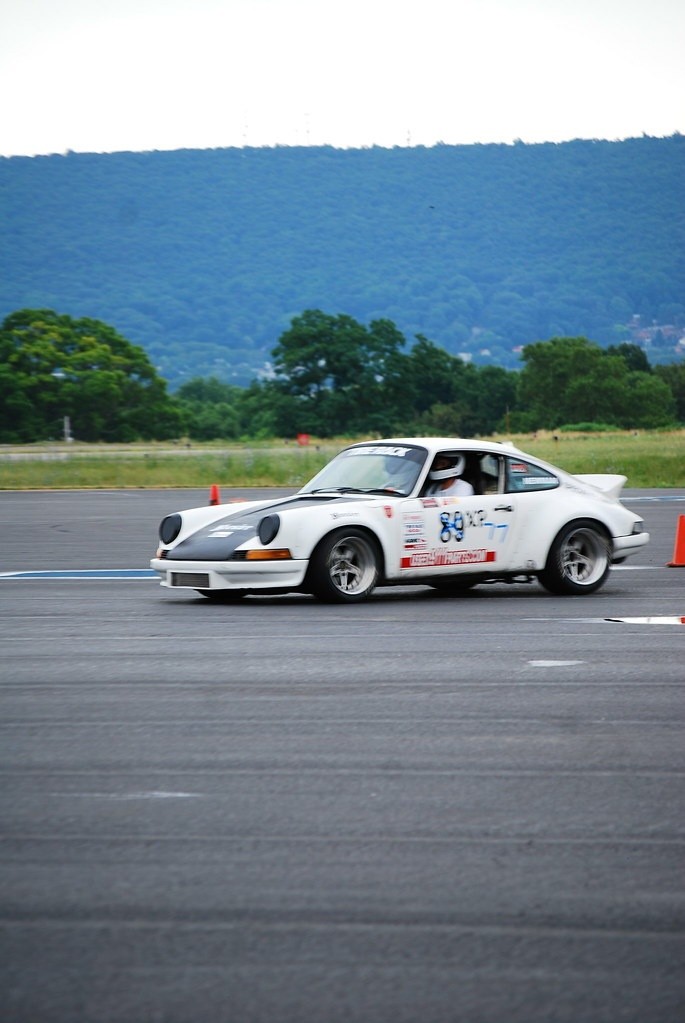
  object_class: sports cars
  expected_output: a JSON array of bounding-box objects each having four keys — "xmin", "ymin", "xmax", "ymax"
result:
[{"xmin": 150, "ymin": 436, "xmax": 650, "ymax": 608}]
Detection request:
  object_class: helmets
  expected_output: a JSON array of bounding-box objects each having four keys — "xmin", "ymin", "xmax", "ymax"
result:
[
  {"xmin": 428, "ymin": 452, "xmax": 465, "ymax": 481},
  {"xmin": 384, "ymin": 451, "xmax": 415, "ymax": 475}
]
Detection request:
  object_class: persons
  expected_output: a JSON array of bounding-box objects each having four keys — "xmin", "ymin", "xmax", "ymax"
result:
[
  {"xmin": 379, "ymin": 449, "xmax": 420, "ymax": 491},
  {"xmin": 426, "ymin": 451, "xmax": 475, "ymax": 496}
]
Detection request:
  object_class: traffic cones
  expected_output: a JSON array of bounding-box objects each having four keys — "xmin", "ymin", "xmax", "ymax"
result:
[
  {"xmin": 664, "ymin": 514, "xmax": 685, "ymax": 567},
  {"xmin": 209, "ymin": 483, "xmax": 220, "ymax": 506}
]
